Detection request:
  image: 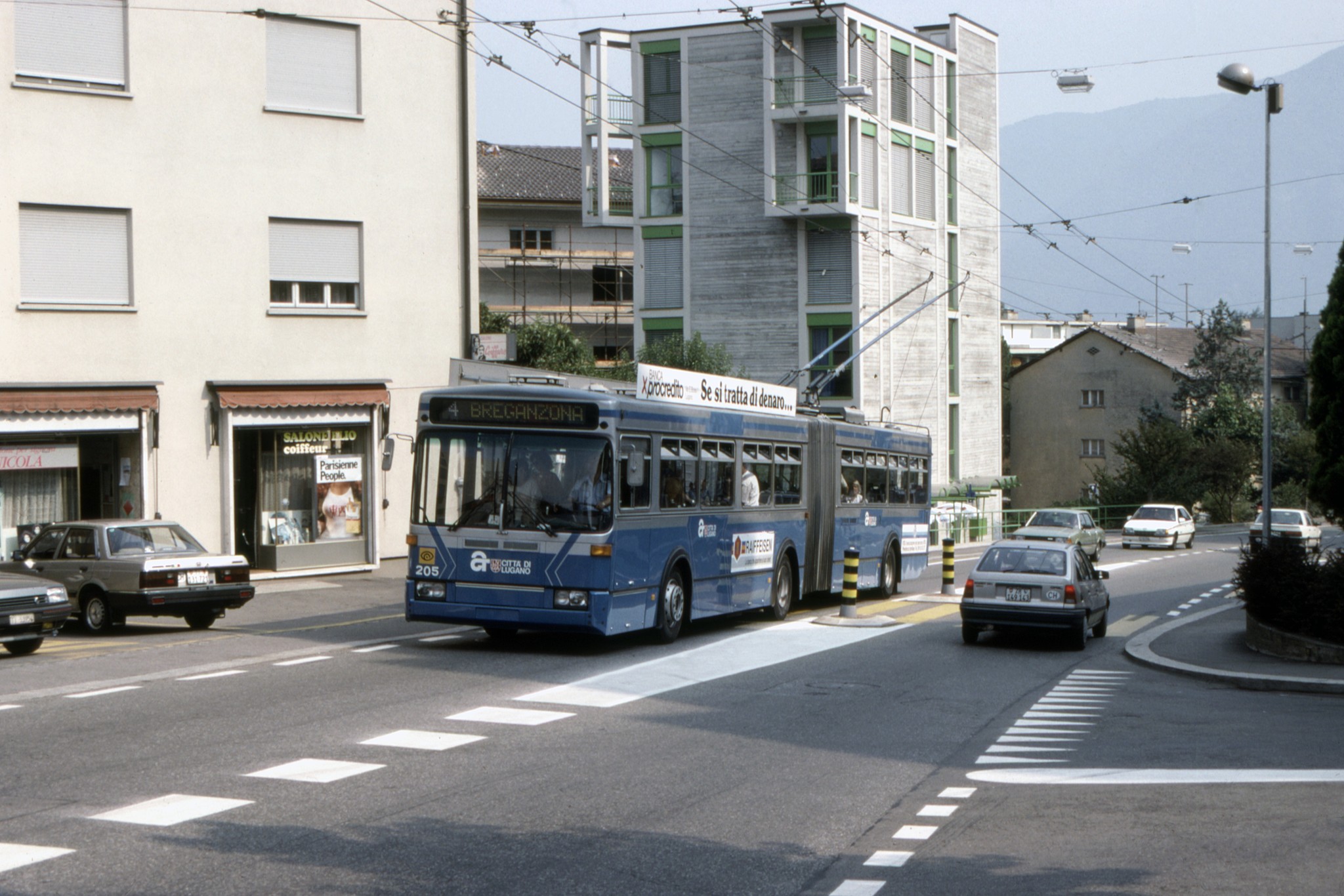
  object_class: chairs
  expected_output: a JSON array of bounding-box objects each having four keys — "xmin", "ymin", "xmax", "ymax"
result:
[
  {"xmin": 118, "ymin": 548, "xmax": 143, "ymax": 554},
  {"xmin": 644, "ymin": 490, "xmax": 801, "ymax": 509},
  {"xmin": 843, "ymin": 483, "xmax": 924, "ymax": 504},
  {"xmin": 1008, "ymin": 552, "xmax": 1028, "ymax": 571},
  {"xmin": 1045, "ymin": 519, "xmax": 1055, "ymax": 526}
]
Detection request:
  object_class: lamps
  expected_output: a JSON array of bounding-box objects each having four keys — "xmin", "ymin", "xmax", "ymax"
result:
[
  {"xmin": 836, "ymin": 86, "xmax": 872, "ymax": 103},
  {"xmin": 1058, "ymin": 75, "xmax": 1095, "ymax": 93}
]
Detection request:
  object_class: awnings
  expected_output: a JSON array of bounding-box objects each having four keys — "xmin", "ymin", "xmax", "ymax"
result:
[
  {"xmin": 0, "ymin": 390, "xmax": 157, "ymax": 413},
  {"xmin": 215, "ymin": 385, "xmax": 389, "ymax": 408}
]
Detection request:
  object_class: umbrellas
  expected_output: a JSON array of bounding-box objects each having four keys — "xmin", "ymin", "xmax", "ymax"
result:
[
  {"xmin": 935, "ymin": 502, "xmax": 981, "ymax": 517},
  {"xmin": 930, "ymin": 507, "xmax": 957, "ymax": 524}
]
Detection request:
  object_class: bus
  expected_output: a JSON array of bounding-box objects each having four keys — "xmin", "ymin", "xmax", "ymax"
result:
[{"xmin": 380, "ymin": 274, "xmax": 972, "ymax": 643}]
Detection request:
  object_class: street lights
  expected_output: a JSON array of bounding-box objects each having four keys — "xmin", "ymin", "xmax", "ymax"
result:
[{"xmin": 1218, "ymin": 63, "xmax": 1283, "ymax": 550}]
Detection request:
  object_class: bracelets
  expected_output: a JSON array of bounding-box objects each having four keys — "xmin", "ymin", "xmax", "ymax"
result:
[
  {"xmin": 601, "ymin": 502, "xmax": 605, "ymax": 506},
  {"xmin": 691, "ymin": 499, "xmax": 695, "ymax": 504}
]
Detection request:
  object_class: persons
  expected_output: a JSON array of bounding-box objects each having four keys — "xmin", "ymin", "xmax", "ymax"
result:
[
  {"xmin": 663, "ymin": 477, "xmax": 695, "ymax": 508},
  {"xmin": 1167, "ymin": 509, "xmax": 1175, "ymax": 520},
  {"xmin": 1068, "ymin": 515, "xmax": 1083, "ymax": 528},
  {"xmin": 530, "ymin": 451, "xmax": 564, "ymax": 511},
  {"xmin": 726, "ymin": 463, "xmax": 759, "ymax": 506},
  {"xmin": 845, "ymin": 479, "xmax": 868, "ymax": 503},
  {"xmin": 689, "ymin": 477, "xmax": 710, "ymax": 507},
  {"xmin": 841, "ymin": 473, "xmax": 848, "ymax": 498},
  {"xmin": 498, "ymin": 458, "xmax": 541, "ymax": 525},
  {"xmin": 318, "ymin": 481, "xmax": 361, "ymax": 537},
  {"xmin": 566, "ymin": 455, "xmax": 611, "ymax": 512}
]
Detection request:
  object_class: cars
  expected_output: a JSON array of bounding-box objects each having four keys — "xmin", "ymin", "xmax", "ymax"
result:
[
  {"xmin": 1011, "ymin": 510, "xmax": 1106, "ymax": 564},
  {"xmin": 0, "ymin": 573, "xmax": 72, "ymax": 655},
  {"xmin": 1248, "ymin": 507, "xmax": 1323, "ymax": 554},
  {"xmin": 959, "ymin": 539, "xmax": 1110, "ymax": 649},
  {"xmin": 1122, "ymin": 505, "xmax": 1197, "ymax": 550},
  {"xmin": 8, "ymin": 516, "xmax": 256, "ymax": 635}
]
